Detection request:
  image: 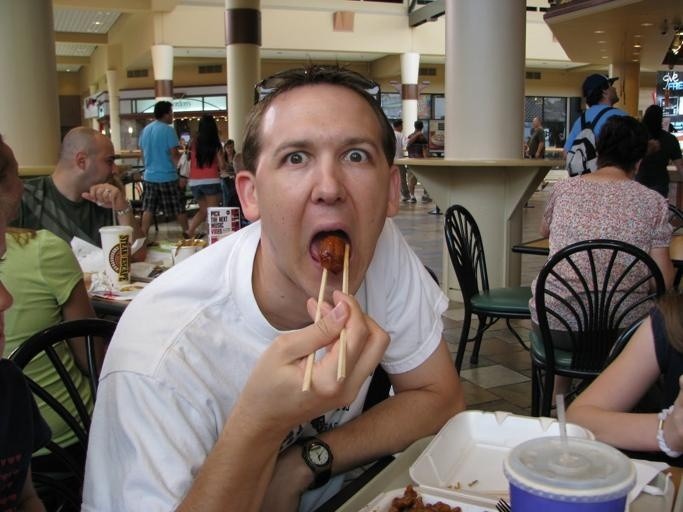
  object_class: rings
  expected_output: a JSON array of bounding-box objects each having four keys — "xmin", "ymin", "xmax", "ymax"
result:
[{"xmin": 104, "ymin": 188, "xmax": 111, "ymax": 192}]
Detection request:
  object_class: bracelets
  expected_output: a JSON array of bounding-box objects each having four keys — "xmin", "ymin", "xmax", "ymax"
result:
[
  {"xmin": 415, "ymin": 134, "xmax": 418, "ymax": 137},
  {"xmin": 657, "ymin": 405, "xmax": 682, "ymax": 458},
  {"xmin": 115, "ymin": 199, "xmax": 133, "ymax": 215}
]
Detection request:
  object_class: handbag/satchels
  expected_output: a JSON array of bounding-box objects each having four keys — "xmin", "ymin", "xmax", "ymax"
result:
[
  {"xmin": 526, "ymin": 147, "xmax": 530, "ymax": 157},
  {"xmin": 177, "ymin": 139, "xmax": 192, "ymax": 179}
]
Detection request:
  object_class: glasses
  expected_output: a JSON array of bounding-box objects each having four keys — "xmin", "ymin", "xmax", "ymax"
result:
[{"xmin": 253, "ymin": 63, "xmax": 383, "ymax": 111}]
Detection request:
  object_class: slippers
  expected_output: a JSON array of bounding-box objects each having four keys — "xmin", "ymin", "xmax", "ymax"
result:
[{"xmin": 541, "ymin": 182, "xmax": 549, "ymax": 192}]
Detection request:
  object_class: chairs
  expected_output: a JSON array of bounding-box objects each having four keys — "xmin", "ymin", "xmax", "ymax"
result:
[
  {"xmin": 530, "ymin": 239, "xmax": 668, "ymax": 420},
  {"xmin": 6, "ymin": 318, "xmax": 119, "ymax": 512},
  {"xmin": 443, "ymin": 205, "xmax": 533, "ymax": 376},
  {"xmin": 124, "ymin": 165, "xmax": 157, "ymax": 233}
]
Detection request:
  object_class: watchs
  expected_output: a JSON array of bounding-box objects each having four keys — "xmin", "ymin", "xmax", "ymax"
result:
[{"xmin": 294, "ymin": 434, "xmax": 334, "ymax": 491}]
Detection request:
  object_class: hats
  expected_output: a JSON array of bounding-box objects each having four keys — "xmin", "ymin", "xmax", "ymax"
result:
[{"xmin": 583, "ymin": 73, "xmax": 619, "ymax": 98}]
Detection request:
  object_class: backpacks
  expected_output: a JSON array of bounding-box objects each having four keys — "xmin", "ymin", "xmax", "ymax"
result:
[{"xmin": 565, "ymin": 106, "xmax": 616, "ymax": 178}]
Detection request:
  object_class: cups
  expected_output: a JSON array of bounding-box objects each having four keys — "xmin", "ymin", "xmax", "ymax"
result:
[
  {"xmin": 98, "ymin": 224, "xmax": 133, "ymax": 283},
  {"xmin": 172, "ymin": 237, "xmax": 207, "ymax": 266},
  {"xmin": 505, "ymin": 435, "xmax": 638, "ymax": 511}
]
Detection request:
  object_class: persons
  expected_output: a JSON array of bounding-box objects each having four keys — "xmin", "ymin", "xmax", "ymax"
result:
[
  {"xmin": 561, "ymin": 75, "xmax": 661, "ymax": 159},
  {"xmin": 219, "ymin": 138, "xmax": 237, "ymax": 208},
  {"xmin": 391, "ymin": 118, "xmax": 424, "ymax": 201},
  {"xmin": 5, "ymin": 126, "xmax": 148, "ymax": 282},
  {"xmin": 528, "ymin": 116, "xmax": 549, "ymax": 192},
  {"xmin": 634, "ymin": 104, "xmax": 683, "ymax": 197},
  {"xmin": 406, "ymin": 121, "xmax": 431, "ymax": 203},
  {"xmin": 0, "ymin": 137, "xmax": 106, "ymax": 468},
  {"xmin": 79, "ymin": 64, "xmax": 468, "ymax": 512},
  {"xmin": 182, "ymin": 114, "xmax": 227, "ymax": 240},
  {"xmin": 137, "ymin": 101, "xmax": 202, "ymax": 246},
  {"xmin": 563, "ymin": 274, "xmax": 682, "ymax": 467},
  {"xmin": 0, "ymin": 206, "xmax": 53, "ymax": 512},
  {"xmin": 528, "ymin": 115, "xmax": 676, "ymax": 418}
]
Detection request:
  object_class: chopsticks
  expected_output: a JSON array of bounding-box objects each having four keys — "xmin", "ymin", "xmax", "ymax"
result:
[{"xmin": 303, "ymin": 243, "xmax": 350, "ymax": 392}]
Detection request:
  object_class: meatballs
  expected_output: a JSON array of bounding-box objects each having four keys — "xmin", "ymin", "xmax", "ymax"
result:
[{"xmin": 319, "ymin": 235, "xmax": 351, "ymax": 272}]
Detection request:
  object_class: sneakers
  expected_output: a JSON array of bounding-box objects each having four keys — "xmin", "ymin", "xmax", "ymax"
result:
[
  {"xmin": 421, "ymin": 196, "xmax": 432, "ymax": 203},
  {"xmin": 407, "ymin": 196, "xmax": 416, "ymax": 203},
  {"xmin": 401, "ymin": 195, "xmax": 411, "ymax": 201}
]
